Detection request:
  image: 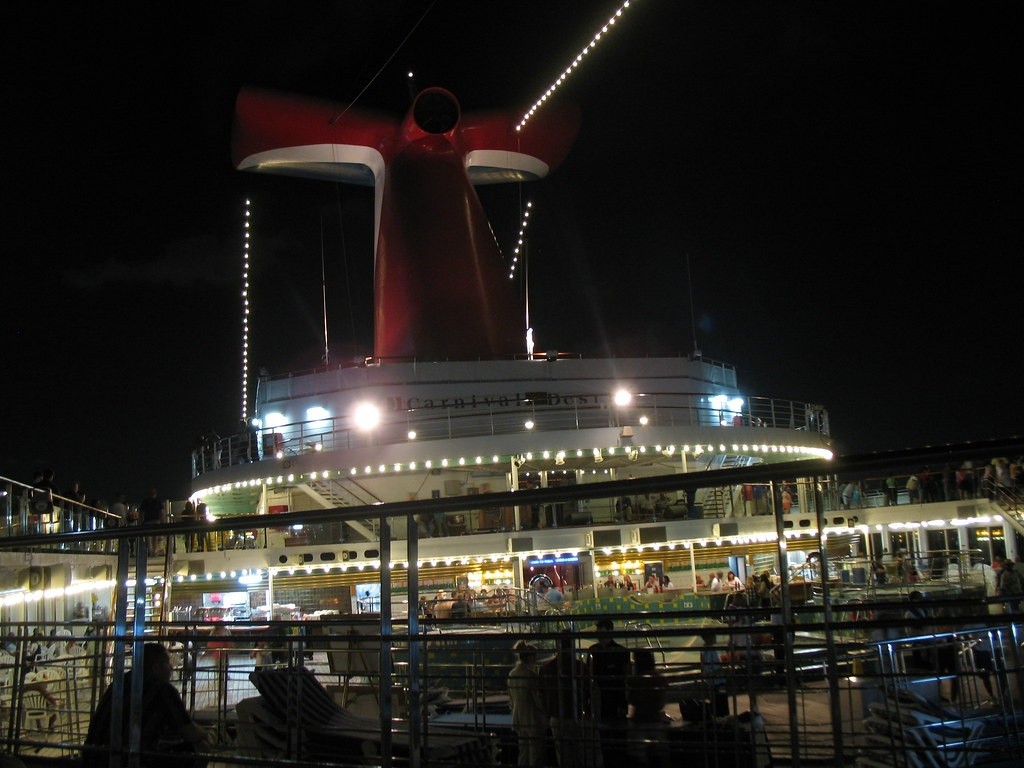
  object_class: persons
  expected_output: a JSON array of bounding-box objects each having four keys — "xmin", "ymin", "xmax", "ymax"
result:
[
  {"xmin": 868, "ymin": 589, "xmax": 1017, "ymax": 710},
  {"xmin": 841, "ymin": 478, "xmax": 867, "ymax": 508},
  {"xmin": 413, "ymin": 551, "xmax": 1024, "ymax": 617},
  {"xmin": 18, "ymin": 469, "xmax": 213, "ymax": 555},
  {"xmin": 525, "ymin": 479, "xmax": 541, "ymax": 530},
  {"xmin": 198, "ymin": 415, "xmax": 261, "ymax": 470},
  {"xmin": 697, "ymin": 628, "xmax": 728, "ymax": 684},
  {"xmin": 1, "ymin": 616, "xmax": 108, "ymax": 722},
  {"xmin": 615, "ymin": 492, "xmax": 672, "ymax": 522},
  {"xmin": 79, "ymin": 642, "xmax": 214, "ymax": 768},
  {"xmin": 501, "ymin": 616, "xmax": 676, "ymax": 768},
  {"xmin": 881, "ymin": 456, "xmax": 1024, "ymax": 506},
  {"xmin": 724, "ymin": 569, "xmax": 791, "ymax": 664},
  {"xmin": 740, "ymin": 477, "xmax": 795, "ymax": 516}
]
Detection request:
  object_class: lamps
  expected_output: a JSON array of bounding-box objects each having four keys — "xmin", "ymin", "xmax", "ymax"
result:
[
  {"xmin": 594, "ymin": 449, "xmax": 603, "ymax": 463},
  {"xmin": 514, "ymin": 454, "xmax": 526, "ymax": 468},
  {"xmin": 629, "ymin": 448, "xmax": 638, "ymax": 461},
  {"xmin": 694, "ymin": 448, "xmax": 704, "ymax": 459},
  {"xmin": 662, "ymin": 447, "xmax": 672, "ymax": 458},
  {"xmin": 555, "ymin": 453, "xmax": 564, "ymax": 465}
]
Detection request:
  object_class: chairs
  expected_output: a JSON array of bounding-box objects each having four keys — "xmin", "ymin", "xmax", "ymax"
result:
[{"xmin": 0, "ymin": 605, "xmax": 1003, "ymax": 768}]
